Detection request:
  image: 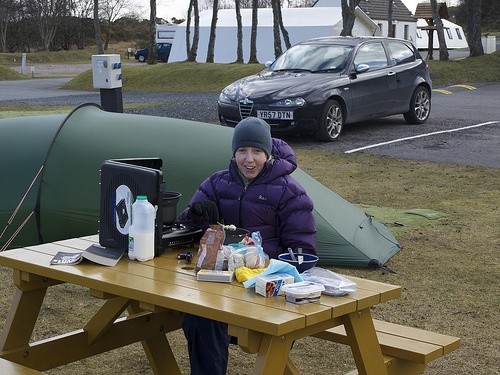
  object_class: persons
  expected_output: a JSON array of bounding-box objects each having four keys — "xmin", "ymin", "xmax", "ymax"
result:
[{"xmin": 172, "ymin": 118, "xmax": 316, "ymax": 375}]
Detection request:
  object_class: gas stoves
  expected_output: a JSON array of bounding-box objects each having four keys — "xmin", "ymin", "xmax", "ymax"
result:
[{"xmin": 162, "ymin": 222, "xmax": 203, "ymax": 249}]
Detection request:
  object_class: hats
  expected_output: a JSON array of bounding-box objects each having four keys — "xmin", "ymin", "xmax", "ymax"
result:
[{"xmin": 231, "ymin": 117, "xmax": 272, "ymax": 158}]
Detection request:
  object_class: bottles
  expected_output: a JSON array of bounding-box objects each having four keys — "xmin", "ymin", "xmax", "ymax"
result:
[{"xmin": 128, "ymin": 195, "xmax": 154, "ymax": 261}]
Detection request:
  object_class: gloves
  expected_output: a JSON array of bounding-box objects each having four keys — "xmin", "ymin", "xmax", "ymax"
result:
[{"xmin": 191, "ymin": 200, "xmax": 220, "ymax": 224}]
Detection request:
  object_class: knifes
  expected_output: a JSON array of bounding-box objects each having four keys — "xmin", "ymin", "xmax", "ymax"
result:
[{"xmin": 298, "ymin": 247, "xmax": 303, "ymax": 264}]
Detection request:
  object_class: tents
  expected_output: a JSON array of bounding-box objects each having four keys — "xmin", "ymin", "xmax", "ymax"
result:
[{"xmin": 0, "ymin": 103, "xmax": 402, "ymax": 267}]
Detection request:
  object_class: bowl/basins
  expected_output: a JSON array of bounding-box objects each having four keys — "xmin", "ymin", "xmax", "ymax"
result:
[
  {"xmin": 283, "ymin": 281, "xmax": 325, "ymax": 304},
  {"xmin": 224, "ymin": 228, "xmax": 250, "ymax": 244},
  {"xmin": 278, "ymin": 252, "xmax": 319, "ymax": 274}
]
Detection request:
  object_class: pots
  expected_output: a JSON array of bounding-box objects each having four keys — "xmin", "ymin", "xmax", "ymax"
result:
[{"xmin": 161, "ymin": 191, "xmax": 182, "ymax": 225}]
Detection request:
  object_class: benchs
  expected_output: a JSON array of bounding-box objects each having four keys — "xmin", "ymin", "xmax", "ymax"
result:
[{"xmin": 314, "ymin": 322, "xmax": 460, "ymax": 374}]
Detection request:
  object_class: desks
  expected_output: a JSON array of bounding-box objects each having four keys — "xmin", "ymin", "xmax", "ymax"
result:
[{"xmin": 0, "ymin": 232, "xmax": 400, "ymax": 375}]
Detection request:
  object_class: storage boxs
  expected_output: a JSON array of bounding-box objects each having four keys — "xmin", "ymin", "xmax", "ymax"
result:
[{"xmin": 255, "ymin": 274, "xmax": 294, "ymax": 298}]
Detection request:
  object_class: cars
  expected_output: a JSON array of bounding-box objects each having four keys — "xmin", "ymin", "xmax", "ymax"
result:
[
  {"xmin": 217, "ymin": 35, "xmax": 434, "ymax": 142},
  {"xmin": 135, "ymin": 42, "xmax": 172, "ymax": 62}
]
